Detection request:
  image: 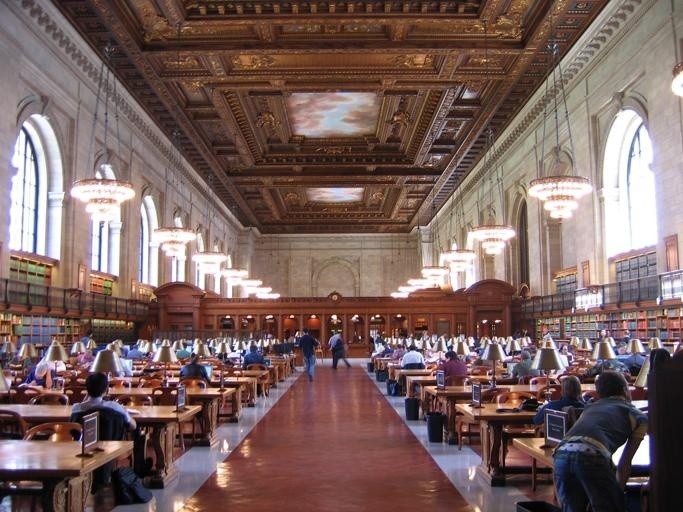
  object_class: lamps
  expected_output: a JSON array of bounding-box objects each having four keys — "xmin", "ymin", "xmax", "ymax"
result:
[{"xmin": 670, "ymin": 62, "xmax": 683, "ymax": 96}]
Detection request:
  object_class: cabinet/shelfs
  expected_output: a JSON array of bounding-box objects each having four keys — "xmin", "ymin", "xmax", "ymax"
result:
[
  {"xmin": 510, "ymin": 298, "xmax": 682, "ymax": 347},
  {"xmin": 0, "ymin": 250, "xmax": 158, "ymax": 354}
]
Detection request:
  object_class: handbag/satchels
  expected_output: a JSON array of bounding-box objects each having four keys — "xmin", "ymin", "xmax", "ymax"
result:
[{"xmin": 111, "ymin": 466, "xmax": 152, "ymax": 504}]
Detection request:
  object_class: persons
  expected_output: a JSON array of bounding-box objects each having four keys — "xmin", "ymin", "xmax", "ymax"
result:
[
  {"xmin": 70, "ymin": 373, "xmax": 138, "ymax": 494},
  {"xmin": 328, "ymin": 331, "xmax": 351, "ymax": 369},
  {"xmin": 299, "ymin": 328, "xmax": 320, "ymax": 382},
  {"xmin": 371, "ymin": 329, "xmax": 652, "ymax": 512},
  {"xmin": 0, "ymin": 329, "xmax": 294, "ymax": 392}
]
